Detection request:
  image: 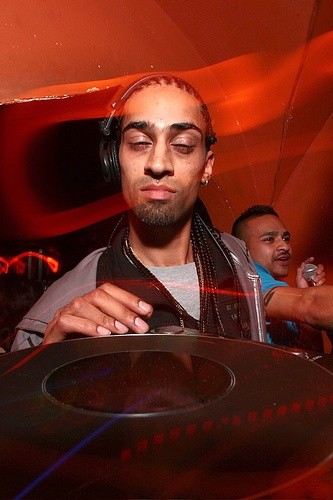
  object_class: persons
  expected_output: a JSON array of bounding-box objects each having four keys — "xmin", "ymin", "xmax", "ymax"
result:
[{"xmin": 9, "ymin": 72, "xmax": 333, "ymax": 354}]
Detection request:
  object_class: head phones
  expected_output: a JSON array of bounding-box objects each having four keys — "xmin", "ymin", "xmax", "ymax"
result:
[{"xmin": 98, "ymin": 73, "xmax": 218, "ymax": 188}]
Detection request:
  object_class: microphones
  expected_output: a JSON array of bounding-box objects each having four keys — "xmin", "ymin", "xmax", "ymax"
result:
[{"xmin": 302, "ymin": 263, "xmax": 320, "ymax": 287}]
